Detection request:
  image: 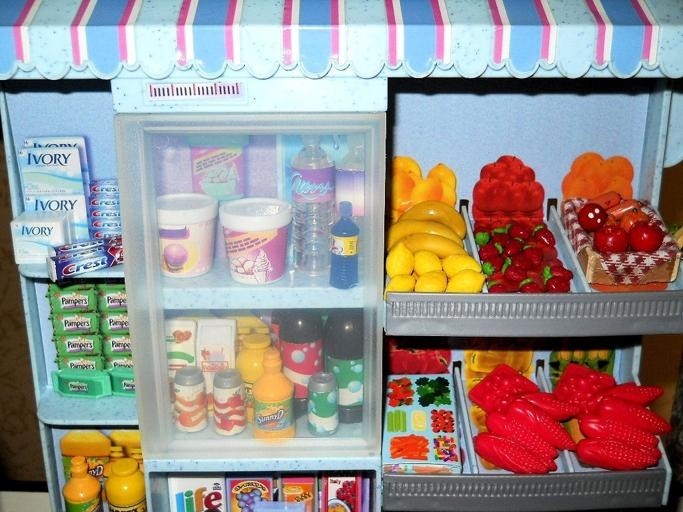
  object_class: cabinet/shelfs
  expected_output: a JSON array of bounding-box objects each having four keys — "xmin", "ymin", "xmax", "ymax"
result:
[{"xmin": 1, "ymin": 77, "xmax": 679, "ymax": 512}]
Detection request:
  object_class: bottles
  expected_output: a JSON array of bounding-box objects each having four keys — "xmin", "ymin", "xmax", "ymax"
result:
[
  {"xmin": 63, "ymin": 456, "xmax": 145, "ymax": 512},
  {"xmin": 178, "ymin": 311, "xmax": 363, "ymax": 439},
  {"xmin": 292, "ymin": 131, "xmax": 360, "ymax": 288}
]
